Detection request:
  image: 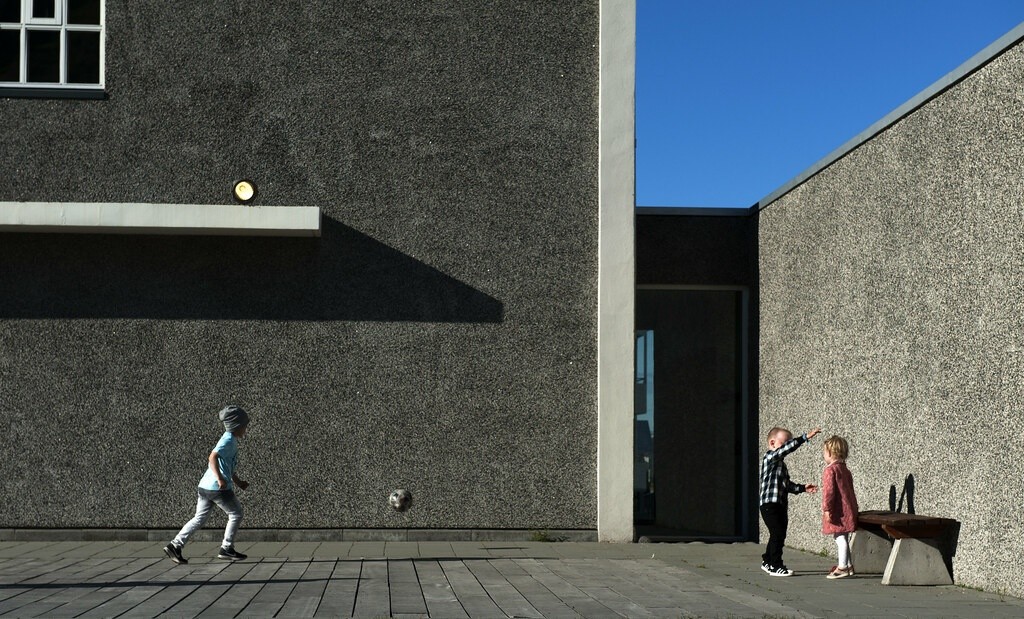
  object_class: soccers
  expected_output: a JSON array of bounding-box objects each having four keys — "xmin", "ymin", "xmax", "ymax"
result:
[{"xmin": 387, "ymin": 487, "xmax": 414, "ymax": 514}]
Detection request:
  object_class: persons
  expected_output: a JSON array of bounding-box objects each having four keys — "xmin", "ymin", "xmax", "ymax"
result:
[
  {"xmin": 163, "ymin": 406, "xmax": 251, "ymax": 564},
  {"xmin": 822, "ymin": 435, "xmax": 858, "ymax": 579},
  {"xmin": 759, "ymin": 425, "xmax": 822, "ymax": 576}
]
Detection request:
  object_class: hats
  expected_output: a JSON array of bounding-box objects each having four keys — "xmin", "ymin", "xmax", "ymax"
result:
[{"xmin": 219, "ymin": 405, "xmax": 250, "ymax": 433}]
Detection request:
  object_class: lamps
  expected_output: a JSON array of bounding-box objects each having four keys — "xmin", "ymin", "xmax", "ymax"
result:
[{"xmin": 231, "ymin": 178, "xmax": 258, "ymax": 207}]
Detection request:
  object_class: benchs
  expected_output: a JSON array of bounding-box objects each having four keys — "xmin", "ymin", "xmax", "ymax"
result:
[{"xmin": 848, "ymin": 509, "xmax": 956, "ymax": 585}]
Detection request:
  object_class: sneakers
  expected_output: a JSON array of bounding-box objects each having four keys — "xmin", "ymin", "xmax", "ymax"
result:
[
  {"xmin": 826, "ymin": 564, "xmax": 855, "ymax": 579},
  {"xmin": 218, "ymin": 548, "xmax": 248, "ymax": 561},
  {"xmin": 760, "ymin": 561, "xmax": 793, "ymax": 577},
  {"xmin": 163, "ymin": 543, "xmax": 188, "ymax": 564}
]
